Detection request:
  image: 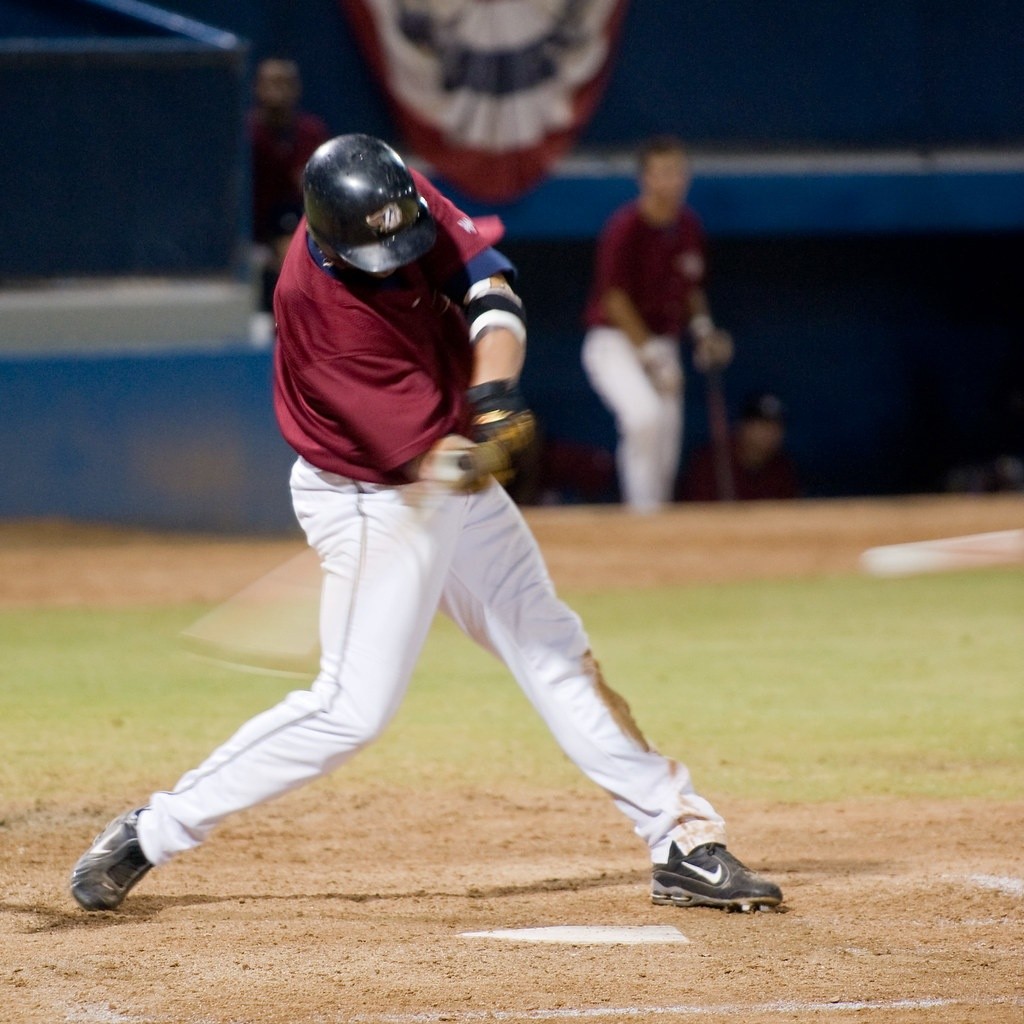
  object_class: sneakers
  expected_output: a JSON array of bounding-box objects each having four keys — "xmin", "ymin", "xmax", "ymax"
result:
[
  {"xmin": 651, "ymin": 840, "xmax": 783, "ymax": 912},
  {"xmin": 69, "ymin": 804, "xmax": 156, "ymax": 912}
]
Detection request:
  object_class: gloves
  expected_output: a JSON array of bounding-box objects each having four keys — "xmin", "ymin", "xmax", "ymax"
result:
[{"xmin": 461, "ymin": 377, "xmax": 536, "ymax": 460}]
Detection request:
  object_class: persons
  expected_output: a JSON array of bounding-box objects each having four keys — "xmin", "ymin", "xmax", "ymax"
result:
[
  {"xmin": 581, "ymin": 141, "xmax": 733, "ymax": 506},
  {"xmin": 249, "ymin": 57, "xmax": 333, "ymax": 313},
  {"xmin": 68, "ymin": 135, "xmax": 786, "ymax": 912},
  {"xmin": 673, "ymin": 394, "xmax": 805, "ymax": 501}
]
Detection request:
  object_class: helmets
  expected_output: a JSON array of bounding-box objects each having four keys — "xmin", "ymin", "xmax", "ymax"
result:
[{"xmin": 301, "ymin": 132, "xmax": 436, "ymax": 273}]
